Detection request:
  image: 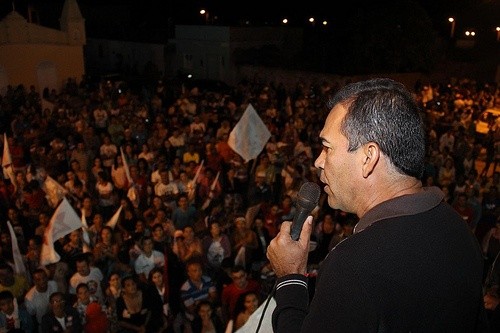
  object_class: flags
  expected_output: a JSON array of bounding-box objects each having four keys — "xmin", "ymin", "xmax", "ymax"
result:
[
  {"xmin": 202, "ymin": 172, "xmax": 220, "ymax": 209},
  {"xmin": 107, "ymin": 205, "xmax": 123, "ymax": 227},
  {"xmin": 120, "ymin": 147, "xmax": 134, "ymax": 185},
  {"xmin": 8, "ymin": 221, "xmax": 26, "ymax": 274},
  {"xmin": 82, "ymin": 209, "xmax": 90, "ymax": 244},
  {"xmin": 227, "ymin": 104, "xmax": 272, "ymax": 162},
  {"xmin": 2, "ymin": 134, "xmax": 17, "ymax": 192},
  {"xmin": 41, "ymin": 199, "xmax": 83, "ymax": 266},
  {"xmin": 190, "ymin": 159, "xmax": 204, "ymax": 195},
  {"xmin": 44, "ymin": 176, "xmax": 68, "ymax": 208}
]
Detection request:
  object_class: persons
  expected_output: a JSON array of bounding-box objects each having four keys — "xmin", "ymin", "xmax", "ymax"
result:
[
  {"xmin": 267, "ymin": 78, "xmax": 367, "ymax": 188},
  {"xmin": 266, "ymin": 78, "xmax": 487, "ymax": 333},
  {"xmin": 0, "ymin": 196, "xmax": 267, "ymax": 333},
  {"xmin": 0, "ymin": 74, "xmax": 267, "ymax": 209},
  {"xmin": 422, "ymin": 173, "xmax": 500, "ymax": 233},
  {"xmin": 412, "ymin": 78, "xmax": 500, "ymax": 173},
  {"xmin": 480, "ymin": 233, "xmax": 500, "ymax": 333},
  {"xmin": 267, "ymin": 183, "xmax": 360, "ymax": 296}
]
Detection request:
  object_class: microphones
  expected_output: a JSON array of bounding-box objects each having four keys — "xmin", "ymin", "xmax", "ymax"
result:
[{"xmin": 289, "ymin": 182, "xmax": 321, "ymax": 241}]
